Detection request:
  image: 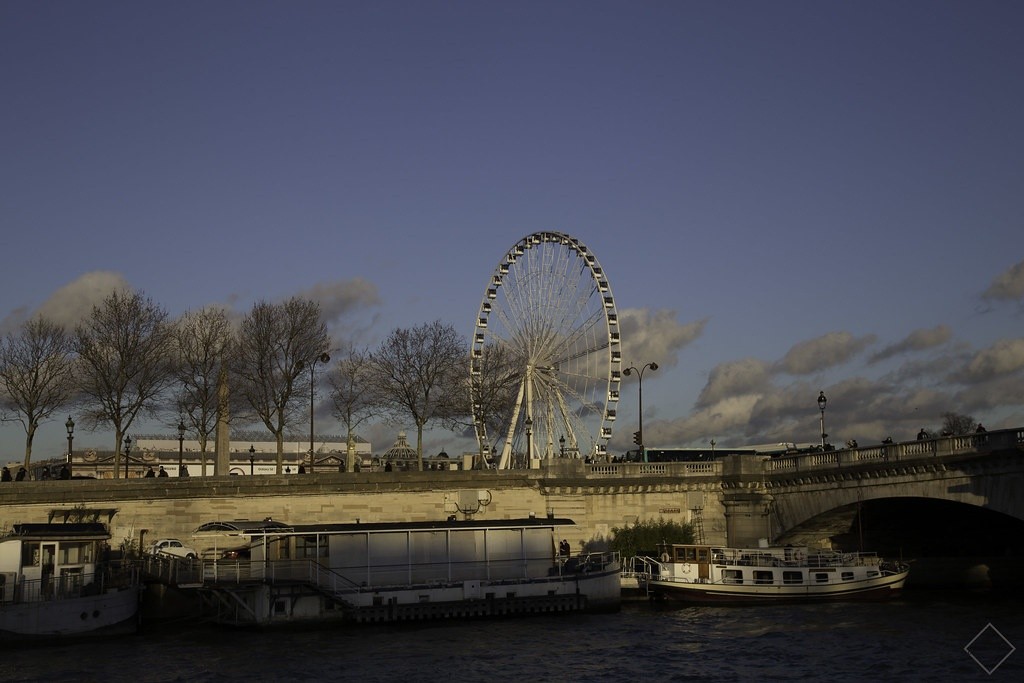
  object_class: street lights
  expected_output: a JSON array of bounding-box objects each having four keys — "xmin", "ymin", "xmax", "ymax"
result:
[
  {"xmin": 623, "ymin": 361, "xmax": 659, "ymax": 462},
  {"xmin": 248, "ymin": 444, "xmax": 255, "ymax": 476},
  {"xmin": 295, "ymin": 352, "xmax": 331, "ymax": 473},
  {"xmin": 524, "ymin": 414, "xmax": 533, "ymax": 470},
  {"xmin": 491, "ymin": 445, "xmax": 498, "ymax": 470},
  {"xmin": 709, "ymin": 438, "xmax": 717, "ymax": 460},
  {"xmin": 816, "ymin": 391, "xmax": 828, "ymax": 452},
  {"xmin": 177, "ymin": 419, "xmax": 187, "ymax": 478},
  {"xmin": 64, "ymin": 414, "xmax": 75, "ymax": 480},
  {"xmin": 123, "ymin": 434, "xmax": 133, "ymax": 478},
  {"xmin": 558, "ymin": 434, "xmax": 567, "ymax": 458}
]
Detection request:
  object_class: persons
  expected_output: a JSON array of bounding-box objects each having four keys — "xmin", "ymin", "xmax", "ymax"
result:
[
  {"xmin": 1, "ymin": 467, "xmax": 11, "ymax": 482},
  {"xmin": 823, "ymin": 444, "xmax": 831, "ymax": 463},
  {"xmin": 298, "ymin": 463, "xmax": 306, "ymax": 474},
  {"xmin": 385, "ymin": 461, "xmax": 392, "ymax": 471},
  {"xmin": 42, "ymin": 466, "xmax": 50, "ymax": 481},
  {"xmin": 585, "ymin": 456, "xmax": 592, "ymax": 463},
  {"xmin": 612, "ymin": 454, "xmax": 624, "ymax": 464},
  {"xmin": 146, "ymin": 467, "xmax": 154, "ymax": 477},
  {"xmin": 917, "ymin": 429, "xmax": 928, "ymax": 452},
  {"xmin": 339, "ymin": 461, "xmax": 345, "ymax": 472},
  {"xmin": 976, "ymin": 423, "xmax": 987, "ymax": 447},
  {"xmin": 159, "ymin": 466, "xmax": 165, "ymax": 477},
  {"xmin": 15, "ymin": 468, "xmax": 27, "ymax": 481},
  {"xmin": 61, "ymin": 466, "xmax": 70, "ymax": 480},
  {"xmin": 560, "ymin": 540, "xmax": 570, "ymax": 558},
  {"xmin": 182, "ymin": 464, "xmax": 189, "ymax": 477},
  {"xmin": 882, "ymin": 437, "xmax": 893, "ymax": 460},
  {"xmin": 852, "ymin": 440, "xmax": 858, "ymax": 448}
]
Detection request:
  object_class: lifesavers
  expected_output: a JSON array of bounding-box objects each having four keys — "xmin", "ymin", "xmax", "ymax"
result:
[
  {"xmin": 682, "ymin": 563, "xmax": 691, "ymax": 574},
  {"xmin": 794, "ymin": 550, "xmax": 804, "ymax": 561},
  {"xmin": 661, "ymin": 553, "xmax": 669, "ymax": 563}
]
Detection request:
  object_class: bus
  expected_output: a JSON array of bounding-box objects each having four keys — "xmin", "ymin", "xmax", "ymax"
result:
[{"xmin": 624, "ymin": 441, "xmax": 836, "ymax": 473}]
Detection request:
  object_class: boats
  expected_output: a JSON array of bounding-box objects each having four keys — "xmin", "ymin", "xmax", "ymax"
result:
[
  {"xmin": 191, "ymin": 507, "xmax": 627, "ymax": 633},
  {"xmin": 0, "ymin": 507, "xmax": 148, "ymax": 641},
  {"xmin": 637, "ymin": 539, "xmax": 912, "ymax": 605}
]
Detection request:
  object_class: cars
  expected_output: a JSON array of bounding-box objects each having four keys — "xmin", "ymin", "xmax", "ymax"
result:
[
  {"xmin": 220, "ymin": 541, "xmax": 252, "ymax": 569},
  {"xmin": 146, "ymin": 537, "xmax": 198, "ymax": 565}
]
